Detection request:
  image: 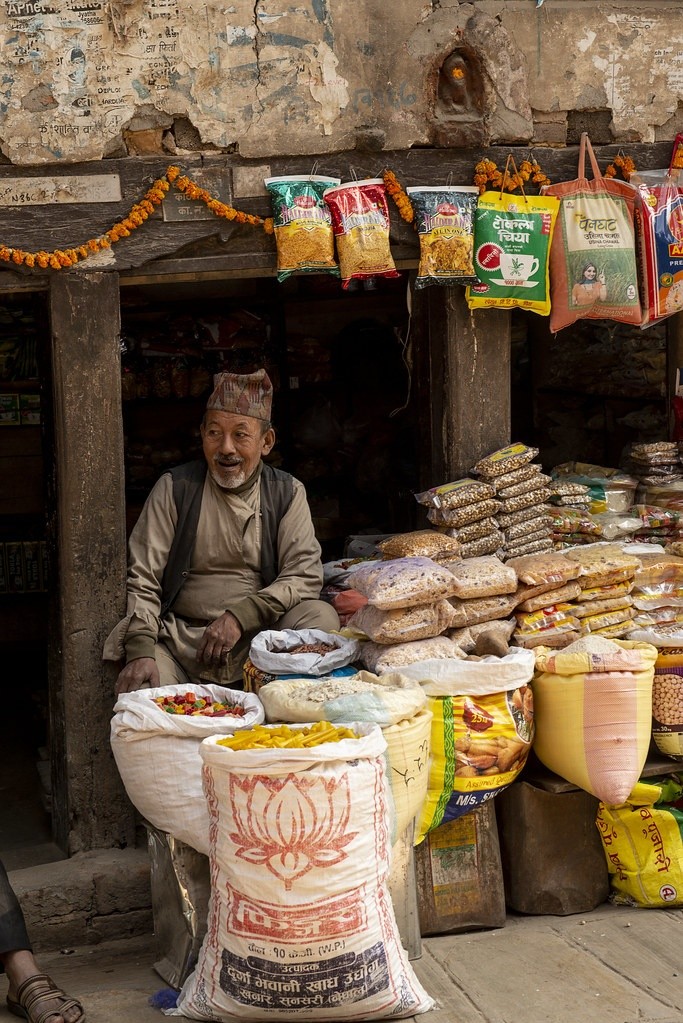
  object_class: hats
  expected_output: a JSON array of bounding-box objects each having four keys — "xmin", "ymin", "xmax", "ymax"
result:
[{"xmin": 206, "ymin": 369, "xmax": 273, "ymax": 423}]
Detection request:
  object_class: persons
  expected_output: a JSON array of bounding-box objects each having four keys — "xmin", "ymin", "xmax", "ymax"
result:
[
  {"xmin": 0, "ymin": 859, "xmax": 87, "ymax": 1023},
  {"xmin": 100, "ymin": 368, "xmax": 340, "ymax": 694}
]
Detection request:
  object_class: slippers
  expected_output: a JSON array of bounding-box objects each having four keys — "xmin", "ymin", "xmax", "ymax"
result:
[{"xmin": 6, "ymin": 973, "xmax": 86, "ymax": 1023}]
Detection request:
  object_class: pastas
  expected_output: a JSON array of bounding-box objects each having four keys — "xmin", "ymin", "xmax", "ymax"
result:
[{"xmin": 217, "ymin": 720, "xmax": 363, "ymax": 751}]
